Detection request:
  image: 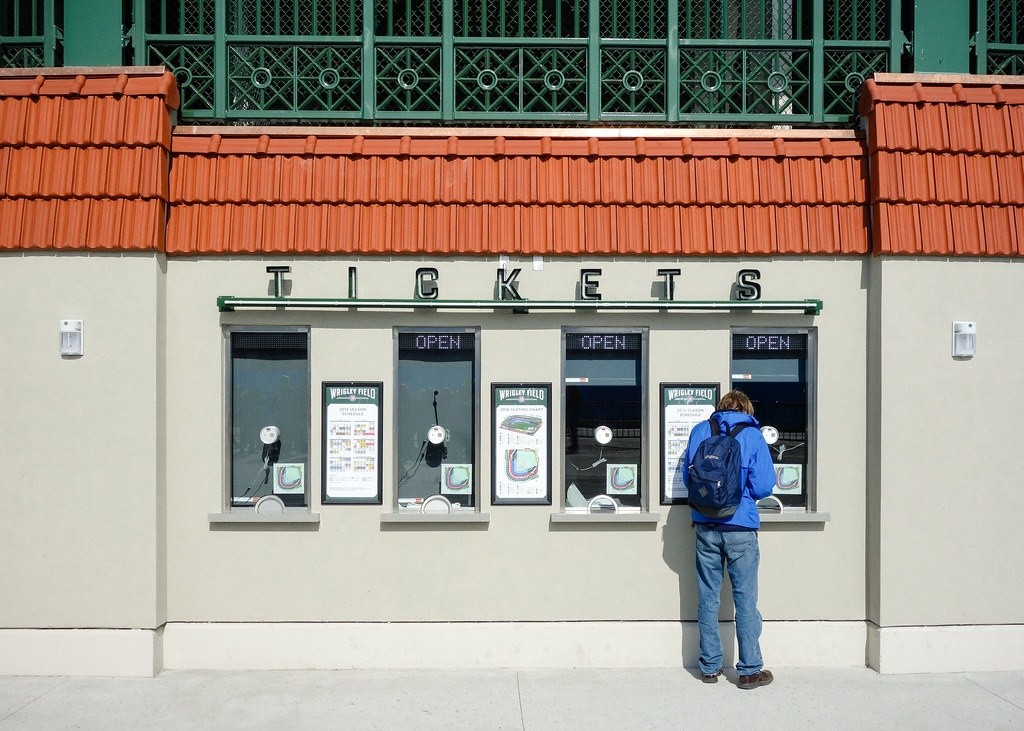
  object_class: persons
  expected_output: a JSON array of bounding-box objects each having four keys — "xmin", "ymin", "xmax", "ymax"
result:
[{"xmin": 683, "ymin": 390, "xmax": 778, "ymax": 690}]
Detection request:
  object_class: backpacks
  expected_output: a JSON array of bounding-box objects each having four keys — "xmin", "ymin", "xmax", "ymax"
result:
[{"xmin": 686, "ymin": 418, "xmax": 757, "ymax": 520}]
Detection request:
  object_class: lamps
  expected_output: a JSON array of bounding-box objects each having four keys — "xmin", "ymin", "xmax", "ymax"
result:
[
  {"xmin": 59, "ymin": 320, "xmax": 84, "ymax": 356},
  {"xmin": 952, "ymin": 321, "xmax": 976, "ymax": 358}
]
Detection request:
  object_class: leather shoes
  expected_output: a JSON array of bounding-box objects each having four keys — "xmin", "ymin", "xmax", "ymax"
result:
[
  {"xmin": 737, "ymin": 670, "xmax": 773, "ymax": 689},
  {"xmin": 702, "ymin": 668, "xmax": 723, "ymax": 683}
]
